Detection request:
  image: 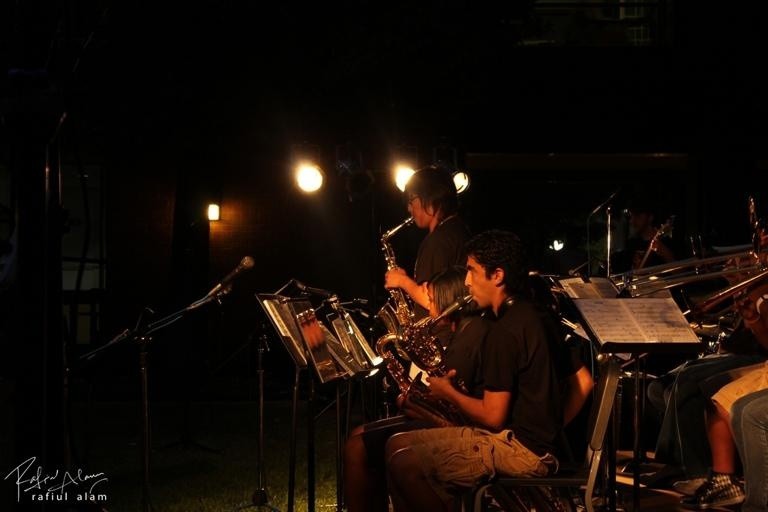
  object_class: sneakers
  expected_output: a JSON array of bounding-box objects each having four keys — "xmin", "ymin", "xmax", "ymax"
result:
[{"xmin": 640, "ymin": 472, "xmax": 747, "ymax": 508}]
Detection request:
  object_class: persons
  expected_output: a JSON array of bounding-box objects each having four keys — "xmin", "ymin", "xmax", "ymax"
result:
[
  {"xmin": 383, "ymin": 227, "xmax": 573, "ymax": 511},
  {"xmin": 612, "ymin": 192, "xmax": 766, "ymax": 512},
  {"xmin": 384, "ymin": 165, "xmax": 471, "ymax": 335},
  {"xmin": 338, "ymin": 261, "xmax": 487, "ymax": 511},
  {"xmin": 508, "ymin": 272, "xmax": 596, "ymax": 468}
]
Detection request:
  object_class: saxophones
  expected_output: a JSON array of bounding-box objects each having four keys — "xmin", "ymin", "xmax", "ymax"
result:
[
  {"xmin": 399, "ymin": 294, "xmax": 470, "ymax": 427},
  {"xmin": 376, "ymin": 316, "xmax": 410, "ymax": 401},
  {"xmin": 381, "ymin": 215, "xmax": 415, "ymax": 359}
]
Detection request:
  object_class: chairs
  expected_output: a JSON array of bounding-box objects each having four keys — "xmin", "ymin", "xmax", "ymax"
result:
[{"xmin": 473, "ymin": 363, "xmax": 622, "ymax": 512}]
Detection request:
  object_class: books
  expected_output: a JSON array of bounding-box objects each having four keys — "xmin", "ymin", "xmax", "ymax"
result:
[
  {"xmin": 262, "ymin": 297, "xmax": 378, "ymax": 384},
  {"xmin": 558, "ymin": 276, "xmax": 701, "ymax": 346}
]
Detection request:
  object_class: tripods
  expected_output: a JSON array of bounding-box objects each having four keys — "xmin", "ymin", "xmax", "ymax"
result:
[
  {"xmin": 77, "ymin": 307, "xmax": 190, "ymax": 512},
  {"xmin": 235, "ymin": 280, "xmax": 286, "ymax": 512}
]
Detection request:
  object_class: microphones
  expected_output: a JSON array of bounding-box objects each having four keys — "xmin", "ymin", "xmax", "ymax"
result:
[{"xmin": 204, "ymin": 256, "xmax": 257, "ymax": 303}]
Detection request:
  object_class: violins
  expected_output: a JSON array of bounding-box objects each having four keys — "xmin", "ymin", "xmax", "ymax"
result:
[{"xmin": 706, "ymin": 267, "xmax": 768, "ymax": 316}]
